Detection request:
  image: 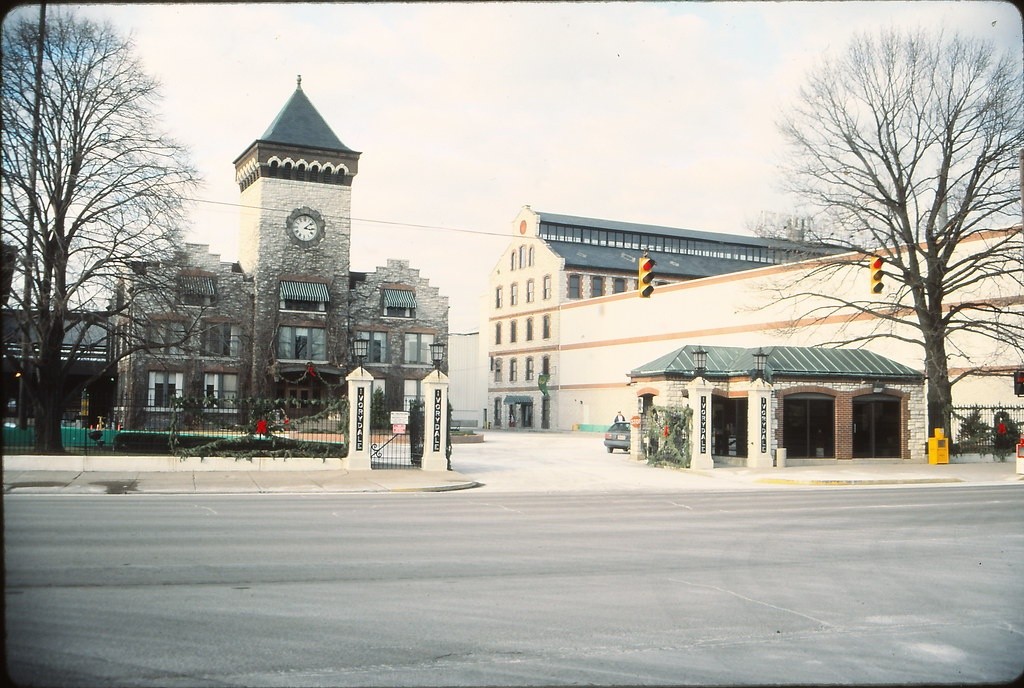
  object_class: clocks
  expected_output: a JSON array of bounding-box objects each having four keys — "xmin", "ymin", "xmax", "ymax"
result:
[{"xmin": 292, "ymin": 213, "xmax": 318, "ymax": 241}]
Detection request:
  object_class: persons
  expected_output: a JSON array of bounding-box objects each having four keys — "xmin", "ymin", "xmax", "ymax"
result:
[{"xmin": 614, "ymin": 409, "xmax": 625, "ymax": 427}]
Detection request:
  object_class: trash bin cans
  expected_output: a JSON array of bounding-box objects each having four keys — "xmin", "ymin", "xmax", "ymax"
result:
[
  {"xmin": 76, "ymin": 415, "xmax": 88, "ymax": 429},
  {"xmin": 776, "ymin": 448, "xmax": 787, "ymax": 468},
  {"xmin": 928, "ymin": 428, "xmax": 949, "ymax": 464},
  {"xmin": 1016, "ymin": 434, "xmax": 1024, "ymax": 480}
]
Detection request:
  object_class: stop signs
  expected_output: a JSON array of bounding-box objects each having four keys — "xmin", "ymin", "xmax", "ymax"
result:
[{"xmin": 631, "ymin": 415, "xmax": 642, "ymax": 429}]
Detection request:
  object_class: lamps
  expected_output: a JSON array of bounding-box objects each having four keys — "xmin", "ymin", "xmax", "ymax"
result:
[
  {"xmin": 691, "ymin": 345, "xmax": 709, "ymax": 377},
  {"xmin": 352, "ymin": 333, "xmax": 370, "ymax": 367},
  {"xmin": 752, "ymin": 346, "xmax": 769, "ymax": 378},
  {"xmin": 428, "ymin": 335, "xmax": 446, "ymax": 370}
]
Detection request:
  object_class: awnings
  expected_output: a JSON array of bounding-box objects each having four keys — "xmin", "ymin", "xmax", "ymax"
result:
[
  {"xmin": 504, "ymin": 396, "xmax": 532, "ymax": 405},
  {"xmin": 280, "ymin": 282, "xmax": 329, "ymax": 302},
  {"xmin": 180, "ymin": 276, "xmax": 215, "ymax": 295},
  {"xmin": 384, "ymin": 290, "xmax": 417, "ymax": 309}
]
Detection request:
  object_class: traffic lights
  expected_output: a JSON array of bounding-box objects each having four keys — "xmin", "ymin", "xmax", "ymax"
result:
[
  {"xmin": 1014, "ymin": 372, "xmax": 1024, "ymax": 394},
  {"xmin": 639, "ymin": 256, "xmax": 656, "ymax": 299},
  {"xmin": 870, "ymin": 255, "xmax": 885, "ymax": 296}
]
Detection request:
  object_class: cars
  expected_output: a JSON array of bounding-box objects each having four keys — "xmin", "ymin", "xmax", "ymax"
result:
[{"xmin": 603, "ymin": 421, "xmax": 631, "ymax": 454}]
